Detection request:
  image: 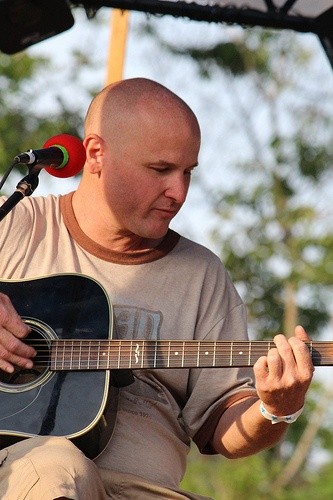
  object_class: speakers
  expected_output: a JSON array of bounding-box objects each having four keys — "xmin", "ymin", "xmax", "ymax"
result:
[{"xmin": 1, "ymin": 0, "xmax": 75, "ymax": 54}]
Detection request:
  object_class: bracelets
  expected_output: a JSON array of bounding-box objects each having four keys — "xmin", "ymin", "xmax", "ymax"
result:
[{"xmin": 261, "ymin": 402, "xmax": 304, "ymax": 425}]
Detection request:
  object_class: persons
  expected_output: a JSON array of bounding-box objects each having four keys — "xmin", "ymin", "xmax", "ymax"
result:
[{"xmin": 1, "ymin": 77, "xmax": 315, "ymax": 500}]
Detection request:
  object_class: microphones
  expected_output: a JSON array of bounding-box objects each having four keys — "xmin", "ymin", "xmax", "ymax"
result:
[{"xmin": 13, "ymin": 135, "xmax": 86, "ymax": 178}]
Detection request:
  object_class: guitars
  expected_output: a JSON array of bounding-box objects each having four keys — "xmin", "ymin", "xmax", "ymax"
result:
[{"xmin": 0, "ymin": 273, "xmax": 332, "ymax": 460}]
